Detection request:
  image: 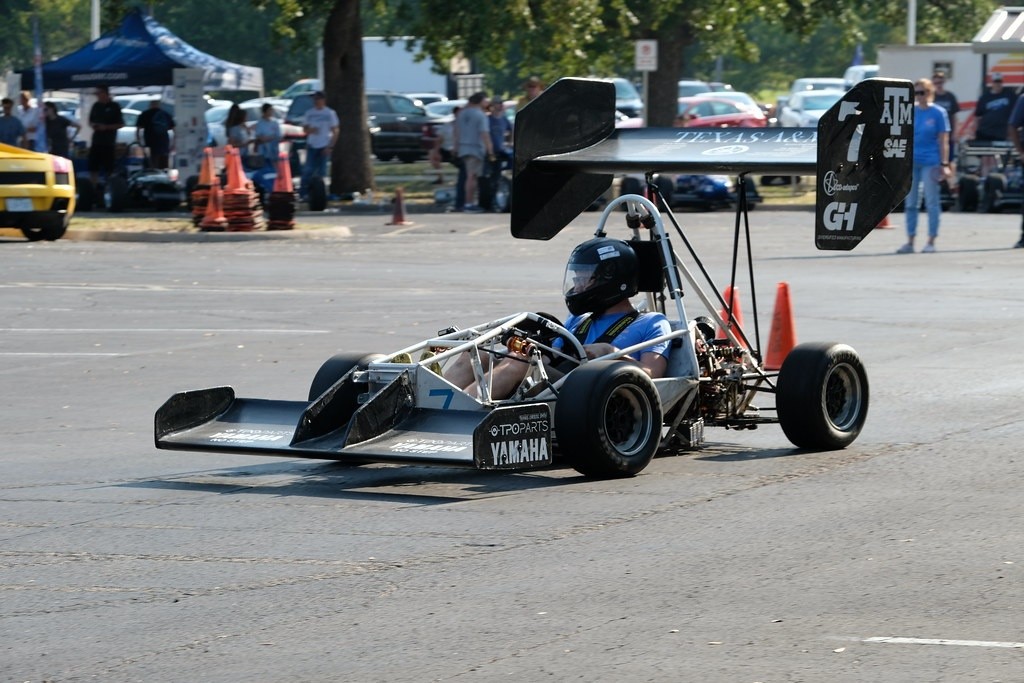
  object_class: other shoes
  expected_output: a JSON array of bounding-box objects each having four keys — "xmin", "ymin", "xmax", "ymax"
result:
[
  {"xmin": 391, "ymin": 353, "xmax": 411, "ymax": 364},
  {"xmin": 921, "ymin": 244, "xmax": 935, "ymax": 252},
  {"xmin": 419, "ymin": 351, "xmax": 442, "ymax": 377},
  {"xmin": 896, "ymin": 244, "xmax": 914, "ymax": 253},
  {"xmin": 465, "ymin": 204, "xmax": 484, "ymax": 213}
]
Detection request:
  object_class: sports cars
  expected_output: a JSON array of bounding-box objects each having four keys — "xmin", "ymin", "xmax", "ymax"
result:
[{"xmin": 0, "ymin": 141, "xmax": 78, "ymax": 240}]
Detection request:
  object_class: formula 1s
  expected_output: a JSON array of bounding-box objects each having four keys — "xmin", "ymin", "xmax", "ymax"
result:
[
  {"xmin": 619, "ymin": 173, "xmax": 764, "ymax": 210},
  {"xmin": 154, "ymin": 76, "xmax": 914, "ymax": 479},
  {"xmin": 890, "ymin": 138, "xmax": 1024, "ymax": 214}
]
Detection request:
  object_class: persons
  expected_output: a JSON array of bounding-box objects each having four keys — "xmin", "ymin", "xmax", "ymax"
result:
[
  {"xmin": 1007, "ymin": 92, "xmax": 1024, "ymax": 248},
  {"xmin": 390, "ymin": 237, "xmax": 672, "ymax": 401},
  {"xmin": 298, "ymin": 90, "xmax": 339, "ymax": 203},
  {"xmin": 135, "ymin": 101, "xmax": 177, "ymax": 168},
  {"xmin": 16, "ymin": 91, "xmax": 40, "ymax": 151},
  {"xmin": 431, "ymin": 91, "xmax": 512, "ymax": 212},
  {"xmin": 43, "ymin": 102, "xmax": 82, "ymax": 200},
  {"xmin": 896, "ymin": 78, "xmax": 951, "ymax": 254},
  {"xmin": 0, "ymin": 98, "xmax": 27, "ymax": 148},
  {"xmin": 974, "ymin": 73, "xmax": 1016, "ymax": 184},
  {"xmin": 87, "ymin": 84, "xmax": 126, "ymax": 208},
  {"xmin": 932, "ymin": 72, "xmax": 961, "ymax": 189},
  {"xmin": 225, "ymin": 103, "xmax": 283, "ymax": 160}
]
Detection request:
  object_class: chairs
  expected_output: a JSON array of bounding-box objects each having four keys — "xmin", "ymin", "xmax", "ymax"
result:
[{"xmin": 669, "ymin": 318, "xmax": 697, "ymax": 375}]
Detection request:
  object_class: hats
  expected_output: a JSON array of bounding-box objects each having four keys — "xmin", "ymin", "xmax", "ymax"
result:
[
  {"xmin": 991, "ymin": 72, "xmax": 1003, "ymax": 82},
  {"xmin": 308, "ymin": 91, "xmax": 327, "ymax": 98}
]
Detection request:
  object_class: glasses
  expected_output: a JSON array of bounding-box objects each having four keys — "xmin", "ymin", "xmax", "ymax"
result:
[
  {"xmin": 571, "ymin": 273, "xmax": 597, "ymax": 285},
  {"xmin": 915, "ymin": 90, "xmax": 925, "ymax": 95}
]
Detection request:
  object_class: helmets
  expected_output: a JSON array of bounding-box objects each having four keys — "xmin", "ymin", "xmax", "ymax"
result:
[{"xmin": 563, "ymin": 237, "xmax": 638, "ymax": 318}]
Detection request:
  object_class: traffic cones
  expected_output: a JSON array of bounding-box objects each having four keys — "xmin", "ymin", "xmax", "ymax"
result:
[
  {"xmin": 191, "ymin": 143, "xmax": 297, "ymax": 232},
  {"xmin": 875, "ymin": 212, "xmax": 897, "ymax": 229},
  {"xmin": 384, "ymin": 187, "xmax": 416, "ymax": 226},
  {"xmin": 714, "ymin": 287, "xmax": 746, "ymax": 368},
  {"xmin": 763, "ymin": 281, "xmax": 797, "ymax": 371}
]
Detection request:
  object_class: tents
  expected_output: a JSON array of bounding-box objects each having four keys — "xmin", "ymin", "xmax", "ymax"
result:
[{"xmin": 21, "ymin": 7, "xmax": 264, "ymax": 98}]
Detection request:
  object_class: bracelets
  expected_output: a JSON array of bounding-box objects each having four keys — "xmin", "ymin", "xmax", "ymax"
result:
[
  {"xmin": 329, "ymin": 146, "xmax": 335, "ymax": 149},
  {"xmin": 488, "ymin": 153, "xmax": 495, "ymax": 156},
  {"xmin": 940, "ymin": 162, "xmax": 949, "ymax": 166}
]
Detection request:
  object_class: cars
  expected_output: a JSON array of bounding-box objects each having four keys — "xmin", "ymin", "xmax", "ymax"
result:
[{"xmin": 0, "ymin": 62, "xmax": 883, "ymax": 164}]
